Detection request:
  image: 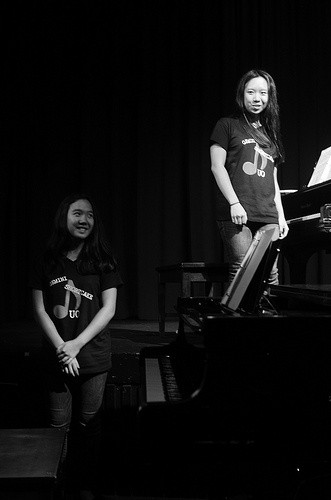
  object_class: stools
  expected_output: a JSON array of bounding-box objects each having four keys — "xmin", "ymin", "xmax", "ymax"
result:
[
  {"xmin": 157, "ymin": 262, "xmax": 229, "ymax": 334},
  {"xmin": 0, "ymin": 427, "xmax": 66, "ymax": 500}
]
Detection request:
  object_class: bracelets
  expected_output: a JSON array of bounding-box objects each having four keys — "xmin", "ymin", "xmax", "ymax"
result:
[{"xmin": 230, "ymin": 202, "xmax": 239, "ymax": 206}]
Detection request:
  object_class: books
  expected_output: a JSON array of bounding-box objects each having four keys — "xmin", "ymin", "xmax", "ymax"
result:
[{"xmin": 221, "ymin": 228, "xmax": 275, "ymax": 310}]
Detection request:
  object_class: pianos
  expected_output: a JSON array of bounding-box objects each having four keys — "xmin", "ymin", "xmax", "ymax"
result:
[{"xmin": 133, "ymin": 215, "xmax": 331, "ymax": 500}]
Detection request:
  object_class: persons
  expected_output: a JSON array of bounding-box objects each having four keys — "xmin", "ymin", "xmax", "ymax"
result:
[
  {"xmin": 29, "ymin": 194, "xmax": 123, "ymax": 464},
  {"xmin": 209, "ymin": 69, "xmax": 289, "ymax": 293}
]
